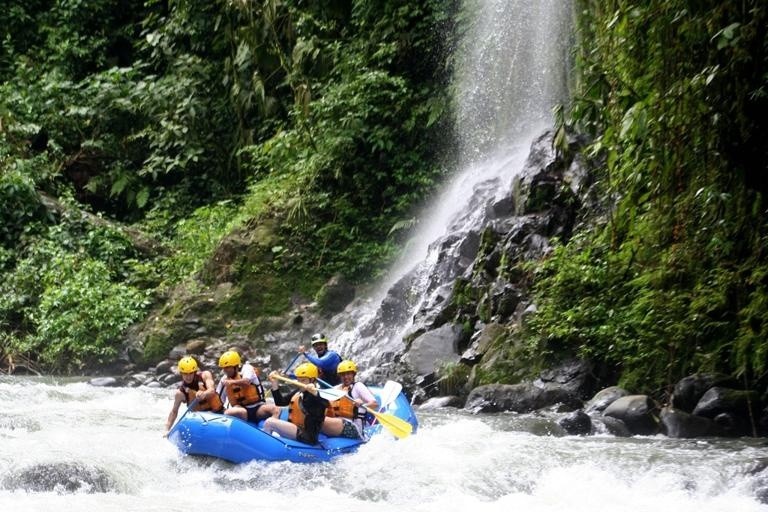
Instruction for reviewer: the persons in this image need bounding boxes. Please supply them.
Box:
[278,333,343,389]
[262,362,331,446]
[316,359,379,439]
[162,354,249,440]
[215,350,283,421]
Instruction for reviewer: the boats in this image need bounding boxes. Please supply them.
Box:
[170,382,418,465]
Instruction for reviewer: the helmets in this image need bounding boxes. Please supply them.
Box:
[294,363,318,384]
[178,355,198,374]
[336,360,358,376]
[218,351,242,369]
[311,333,328,345]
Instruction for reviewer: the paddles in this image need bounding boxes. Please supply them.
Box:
[273,373,348,402]
[315,377,413,441]
